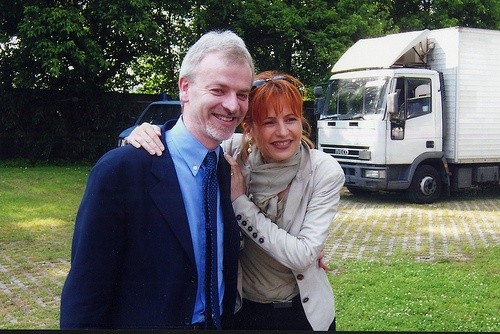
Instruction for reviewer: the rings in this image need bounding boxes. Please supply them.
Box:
[231,172,233,175]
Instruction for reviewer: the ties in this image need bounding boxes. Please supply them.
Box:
[200,151,221,332]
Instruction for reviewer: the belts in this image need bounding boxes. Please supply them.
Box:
[242,296,301,309]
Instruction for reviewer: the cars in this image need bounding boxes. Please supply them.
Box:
[116,101,182,148]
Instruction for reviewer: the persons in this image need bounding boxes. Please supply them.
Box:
[123,70,345,332]
[59,30,330,331]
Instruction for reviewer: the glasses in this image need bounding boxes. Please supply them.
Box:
[250,75,304,98]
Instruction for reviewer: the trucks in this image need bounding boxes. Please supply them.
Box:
[314,26,500,204]
